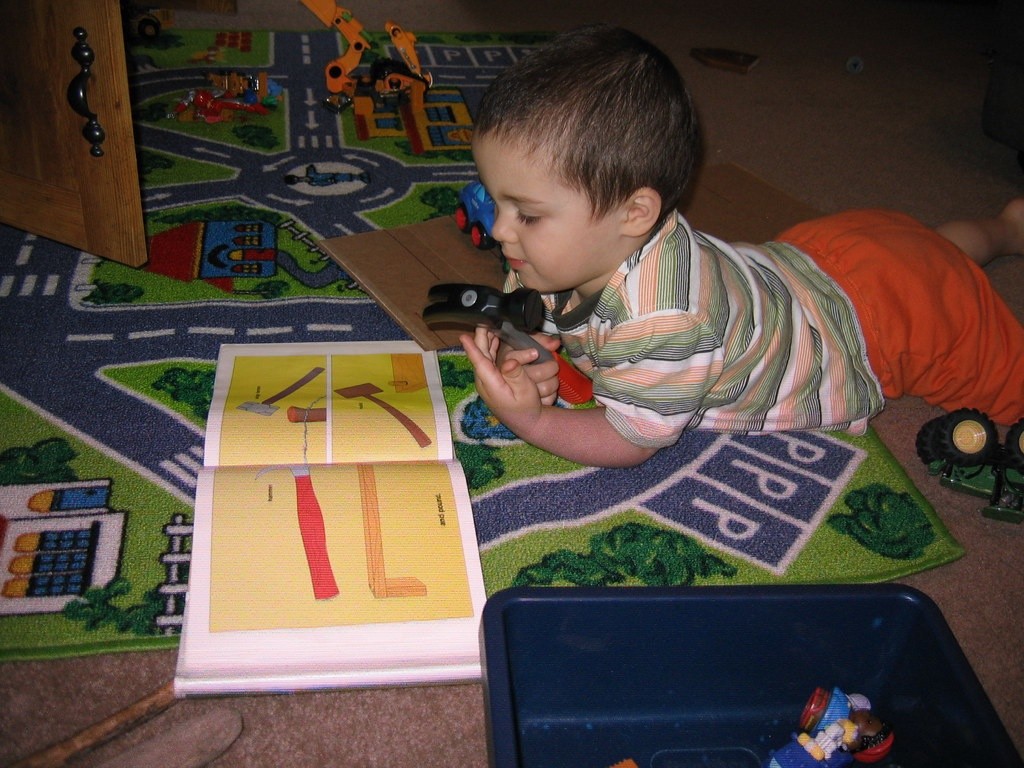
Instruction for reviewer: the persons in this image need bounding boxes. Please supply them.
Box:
[459,23,1024,471]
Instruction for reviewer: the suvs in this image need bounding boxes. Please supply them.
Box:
[915,407,1024,525]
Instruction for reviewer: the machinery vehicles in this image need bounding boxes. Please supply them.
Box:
[299,0,434,114]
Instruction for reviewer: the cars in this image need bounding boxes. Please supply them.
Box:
[456,179,498,250]
[128,7,176,40]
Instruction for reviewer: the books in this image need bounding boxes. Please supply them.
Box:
[175,340,490,698]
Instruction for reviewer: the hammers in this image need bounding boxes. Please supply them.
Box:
[423,282,597,404]
